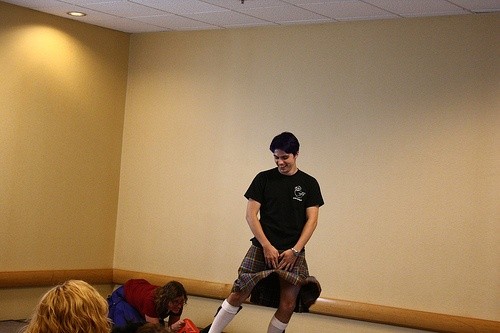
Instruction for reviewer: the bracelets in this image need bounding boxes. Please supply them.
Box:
[169,324,176,332]
[290,247,300,254]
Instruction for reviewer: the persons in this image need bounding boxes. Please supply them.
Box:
[106,274,188,333]
[20,279,113,333]
[207,131,325,333]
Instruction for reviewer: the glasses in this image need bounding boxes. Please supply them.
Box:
[171,299,185,306]
